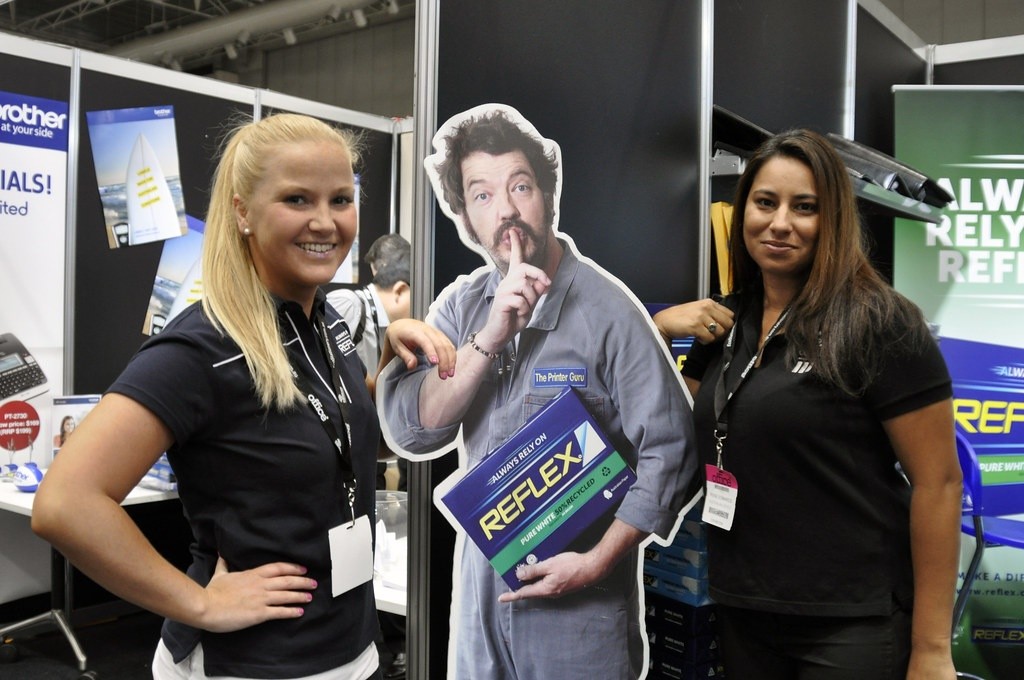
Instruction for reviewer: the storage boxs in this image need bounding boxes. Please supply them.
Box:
[441,385,635,592]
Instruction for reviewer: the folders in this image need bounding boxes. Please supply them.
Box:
[827,130,954,208]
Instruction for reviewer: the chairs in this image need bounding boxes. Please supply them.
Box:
[952,429,1024,639]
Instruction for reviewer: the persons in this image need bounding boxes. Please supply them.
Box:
[384,111,699,679]
[324,232,410,391]
[31,114,456,680]
[652,128,963,679]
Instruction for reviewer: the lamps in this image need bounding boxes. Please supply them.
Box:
[283,28,297,44]
[325,4,341,23]
[384,0,400,16]
[224,42,238,59]
[235,28,252,48]
[352,8,367,28]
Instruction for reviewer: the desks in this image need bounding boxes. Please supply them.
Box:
[0,467,180,680]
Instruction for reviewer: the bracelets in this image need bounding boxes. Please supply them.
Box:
[467,332,506,359]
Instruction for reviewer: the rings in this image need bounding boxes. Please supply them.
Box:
[708,321,717,332]
